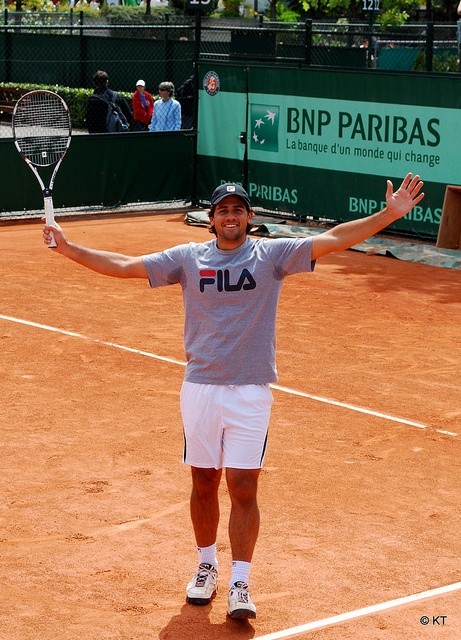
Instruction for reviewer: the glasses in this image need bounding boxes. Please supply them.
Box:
[158,88,165,92]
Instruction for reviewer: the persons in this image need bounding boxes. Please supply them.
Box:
[131,79,154,131]
[360,39,375,61]
[40,171,431,620]
[149,81,182,131]
[90,0,100,11]
[76,0,89,9]
[86,70,131,209]
[140,0,148,7]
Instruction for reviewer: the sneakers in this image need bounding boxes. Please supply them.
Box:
[227,581,256,619]
[186,563,219,605]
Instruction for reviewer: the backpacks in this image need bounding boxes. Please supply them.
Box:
[90,91,130,132]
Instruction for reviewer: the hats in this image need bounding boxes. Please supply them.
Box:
[136,80,145,87]
[210,183,250,205]
